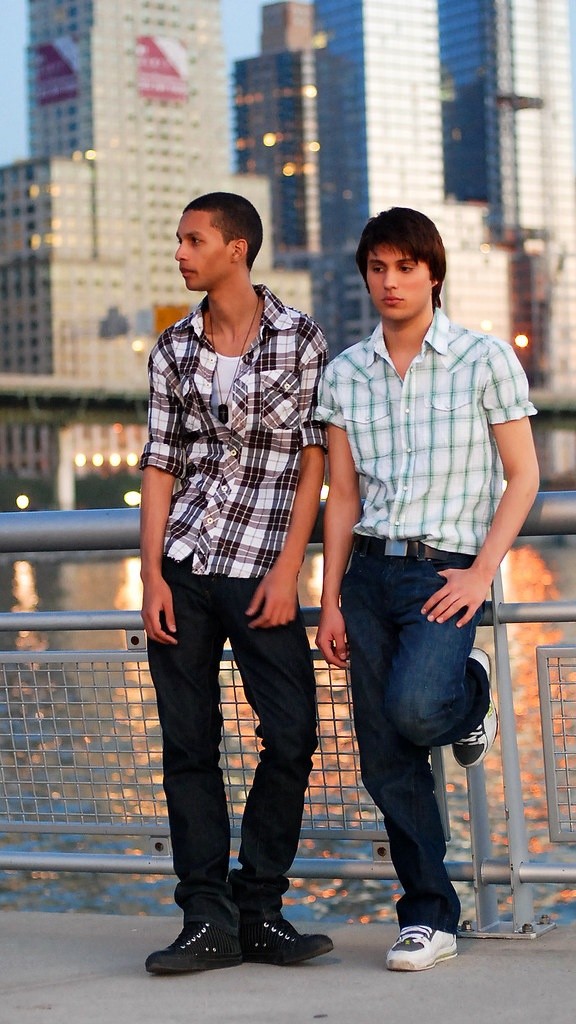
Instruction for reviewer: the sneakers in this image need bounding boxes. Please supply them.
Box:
[145,915,241,973]
[385,925,459,972]
[450,648,500,768]
[241,914,332,966]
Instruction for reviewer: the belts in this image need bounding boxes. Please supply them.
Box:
[355,535,449,561]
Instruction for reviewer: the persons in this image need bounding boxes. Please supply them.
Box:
[138,192,335,978]
[315,208,540,970]
